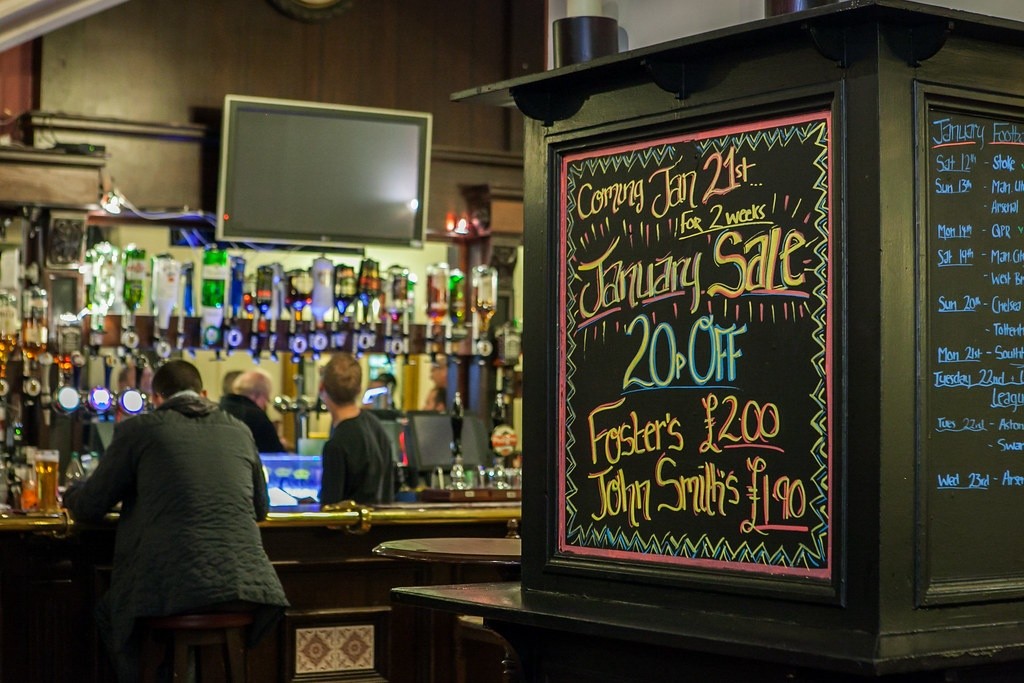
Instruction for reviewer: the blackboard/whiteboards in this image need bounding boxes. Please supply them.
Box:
[548,98,843,596]
[912,81,1024,609]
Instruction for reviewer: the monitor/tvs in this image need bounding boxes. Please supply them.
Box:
[215,95,435,249]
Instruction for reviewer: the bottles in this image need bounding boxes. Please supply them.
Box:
[450,457,465,488]
[86,449,101,479]
[474,264,499,340]
[494,459,505,488]
[21,290,47,367]
[493,393,506,417]
[0,292,17,376]
[155,250,467,332]
[452,392,464,416]
[62,451,84,487]
[89,240,120,333]
[125,245,145,334]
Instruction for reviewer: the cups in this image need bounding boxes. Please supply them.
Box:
[36,451,60,512]
[553,16,620,70]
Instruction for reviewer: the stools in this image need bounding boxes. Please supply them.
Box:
[165,612,254,683]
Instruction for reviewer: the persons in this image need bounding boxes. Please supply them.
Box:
[114,362,155,424]
[63,358,292,683]
[218,366,286,453]
[423,384,462,443]
[367,372,398,410]
[316,353,394,506]
